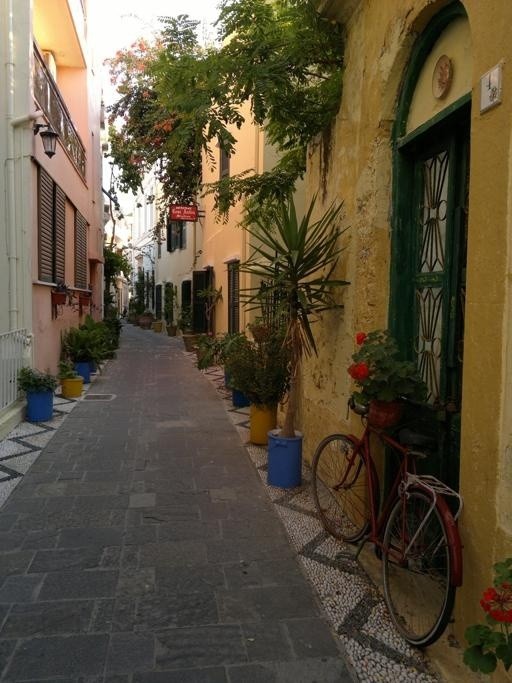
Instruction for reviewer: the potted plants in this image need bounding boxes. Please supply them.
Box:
[19,366,59,422]
[50,279,222,399]
[220,188,352,490]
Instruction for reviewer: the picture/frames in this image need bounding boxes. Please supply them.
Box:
[478,60,503,116]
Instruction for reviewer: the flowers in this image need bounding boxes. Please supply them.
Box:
[345,327,434,403]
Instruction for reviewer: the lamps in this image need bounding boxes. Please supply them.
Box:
[33,121,60,158]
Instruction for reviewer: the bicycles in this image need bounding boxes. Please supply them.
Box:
[311,390,468,652]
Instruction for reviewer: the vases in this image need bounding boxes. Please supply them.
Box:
[366,397,402,430]
